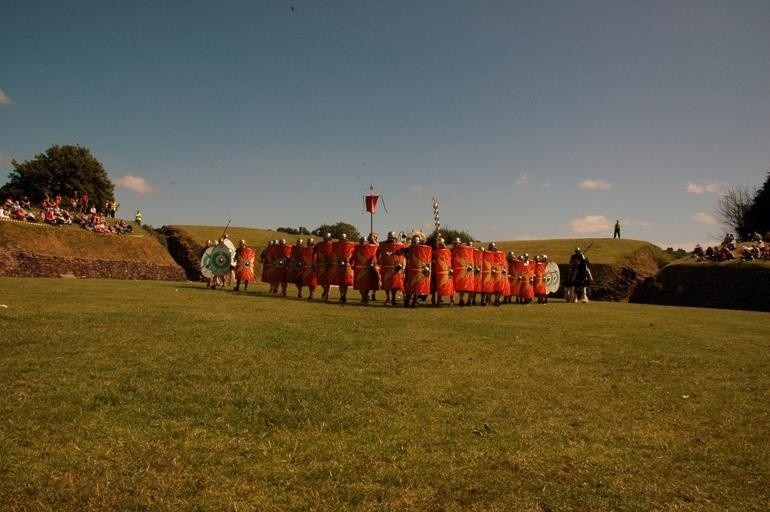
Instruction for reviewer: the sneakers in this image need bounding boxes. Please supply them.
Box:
[207,284,224,289]
[502,298,549,304]
[234,286,248,291]
[383,298,419,307]
[269,288,287,297]
[298,294,313,299]
[338,296,348,302]
[361,294,376,304]
[431,300,455,308]
[459,300,501,307]
[322,292,329,300]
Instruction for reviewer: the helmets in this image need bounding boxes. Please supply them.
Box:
[436,238,445,244]
[388,232,396,238]
[508,251,547,261]
[488,242,497,249]
[324,232,331,238]
[296,239,303,243]
[359,236,368,242]
[575,247,581,252]
[453,237,474,246]
[269,239,286,245]
[205,235,247,246]
[412,236,419,243]
[339,233,347,238]
[307,238,315,243]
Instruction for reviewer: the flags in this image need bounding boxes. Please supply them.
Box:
[363,194,381,215]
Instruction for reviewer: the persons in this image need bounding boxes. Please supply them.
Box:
[258,231,552,309]
[232,239,255,291]
[2,189,133,236]
[134,209,143,228]
[222,232,238,286]
[212,239,222,286]
[198,238,219,287]
[210,237,230,290]
[613,219,621,239]
[691,229,770,264]
[568,247,594,284]
